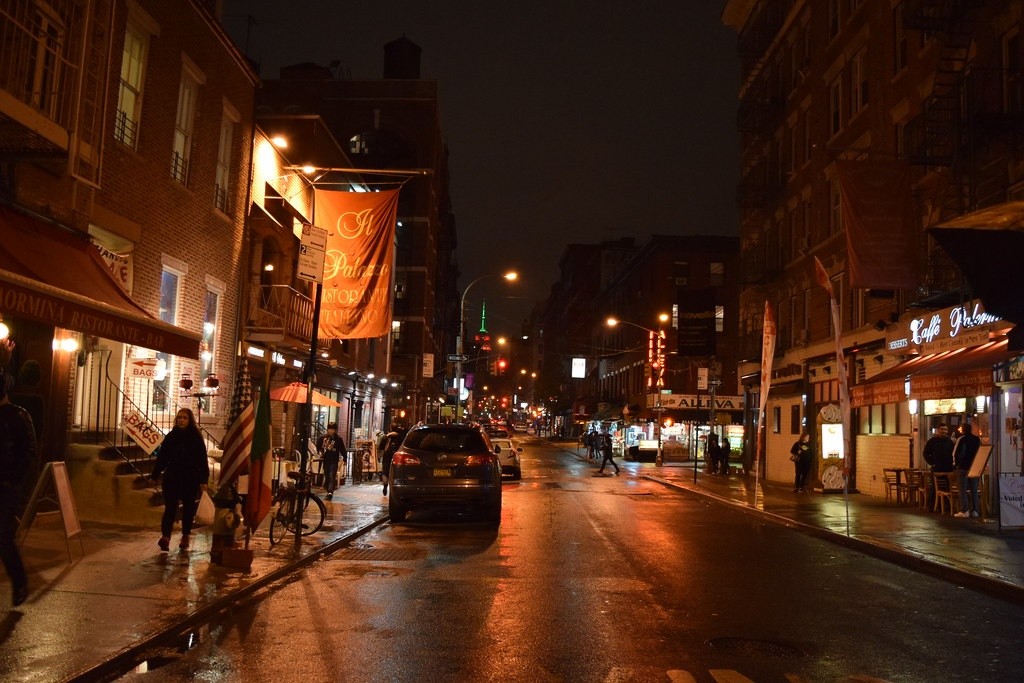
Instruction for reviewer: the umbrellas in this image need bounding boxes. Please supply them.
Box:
[270,381,343,407]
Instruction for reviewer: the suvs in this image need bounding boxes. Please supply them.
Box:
[386,420,504,526]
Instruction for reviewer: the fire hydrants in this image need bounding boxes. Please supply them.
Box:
[209,481,249,566]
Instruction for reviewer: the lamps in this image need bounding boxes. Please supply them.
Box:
[874,319,888,331]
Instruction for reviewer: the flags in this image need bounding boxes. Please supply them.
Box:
[218,356,273,534]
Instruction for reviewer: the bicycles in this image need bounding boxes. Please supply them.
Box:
[266,459,327,545]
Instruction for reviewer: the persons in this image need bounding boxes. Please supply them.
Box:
[149,407,209,552]
[723,438,730,472]
[13,360,45,438]
[583,430,602,459]
[0,375,40,606]
[597,437,620,474]
[790,433,812,492]
[379,422,399,496]
[952,423,982,517]
[561,426,565,438]
[709,439,721,474]
[923,423,955,513]
[317,422,348,500]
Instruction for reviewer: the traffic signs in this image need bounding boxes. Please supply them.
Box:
[297,222,332,292]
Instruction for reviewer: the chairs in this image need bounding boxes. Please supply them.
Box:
[881,467,988,515]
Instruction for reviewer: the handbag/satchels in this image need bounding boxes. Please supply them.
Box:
[790,442,800,462]
[194,491,215,524]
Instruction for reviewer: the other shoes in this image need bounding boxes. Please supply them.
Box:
[383,483,388,496]
[792,488,797,492]
[158,534,169,551]
[800,489,806,492]
[179,536,190,548]
[615,469,620,474]
[971,510,978,517]
[597,470,603,473]
[325,491,332,500]
[13,580,28,604]
[954,510,969,518]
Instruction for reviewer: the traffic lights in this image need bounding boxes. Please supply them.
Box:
[499,360,506,376]
[447,353,470,365]
[489,361,497,377]
[707,379,724,386]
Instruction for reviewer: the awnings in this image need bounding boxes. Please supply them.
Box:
[849,335,1022,408]
[0,204,203,361]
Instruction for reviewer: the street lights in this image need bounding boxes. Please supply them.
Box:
[605,315,666,466]
[452,269,521,420]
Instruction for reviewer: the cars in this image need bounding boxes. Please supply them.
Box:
[515,421,529,432]
[488,438,527,479]
[482,418,512,440]
